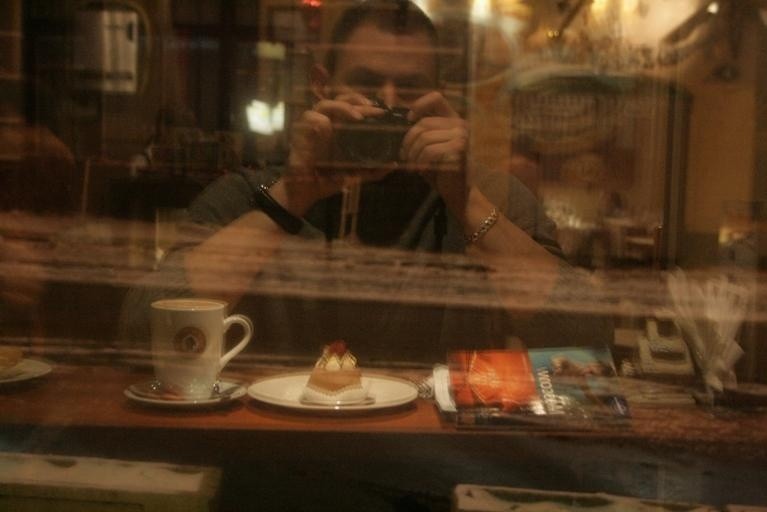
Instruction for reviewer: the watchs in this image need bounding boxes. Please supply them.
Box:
[249,184,304,235]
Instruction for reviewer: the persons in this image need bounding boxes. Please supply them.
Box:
[118,0,615,363]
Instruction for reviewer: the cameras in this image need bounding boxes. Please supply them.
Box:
[317,107,462,173]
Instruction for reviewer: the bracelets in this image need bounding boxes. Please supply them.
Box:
[469,207,499,247]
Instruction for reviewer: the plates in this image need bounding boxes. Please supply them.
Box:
[248,370,420,414]
[1,347,54,385]
[125,382,248,407]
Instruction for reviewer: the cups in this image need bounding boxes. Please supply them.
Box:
[147,297,254,401]
